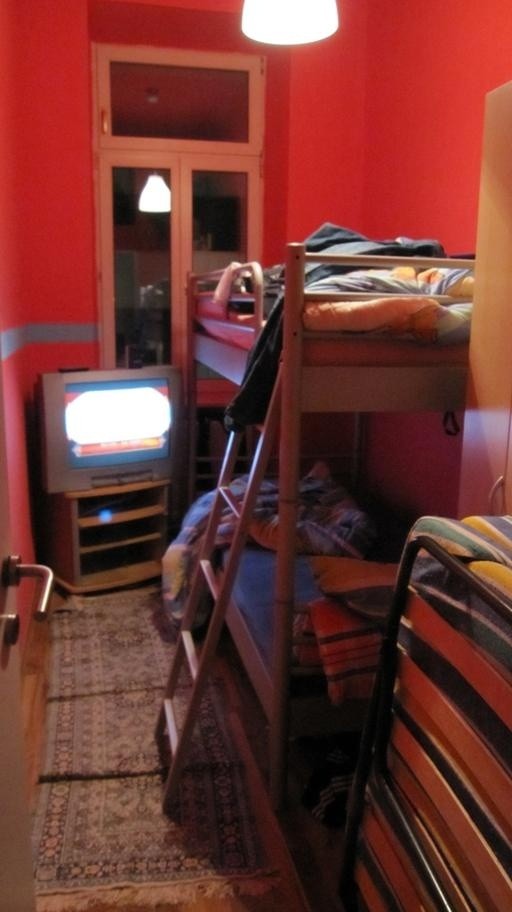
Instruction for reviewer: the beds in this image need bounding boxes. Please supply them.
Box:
[189,254,476,413]
[183,456,407,809]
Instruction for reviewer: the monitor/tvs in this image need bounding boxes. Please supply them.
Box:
[39,366,188,493]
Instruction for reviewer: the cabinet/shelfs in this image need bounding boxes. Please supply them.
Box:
[51,480,170,595]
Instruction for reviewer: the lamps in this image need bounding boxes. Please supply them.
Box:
[237,1,344,46]
[137,168,174,212]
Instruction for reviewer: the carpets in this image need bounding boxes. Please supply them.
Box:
[25,590,276,899]
